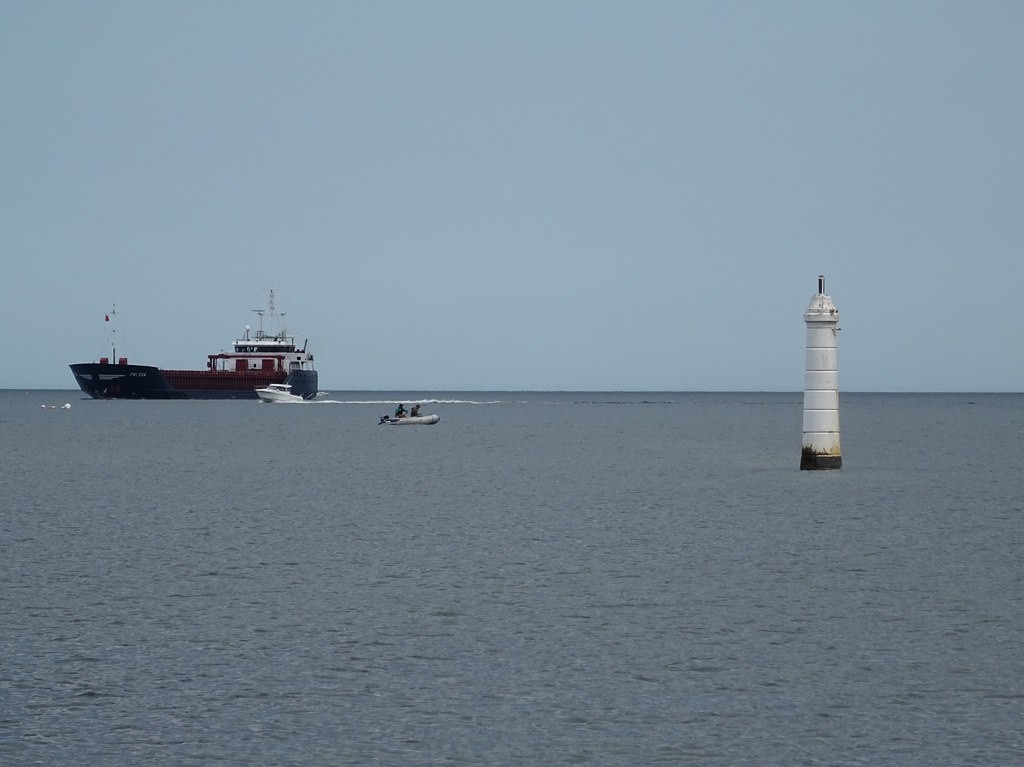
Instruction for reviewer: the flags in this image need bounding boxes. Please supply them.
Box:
[105,314,109,322]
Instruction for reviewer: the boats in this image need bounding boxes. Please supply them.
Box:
[67,288,317,402]
[255,382,304,403]
[378,414,440,425]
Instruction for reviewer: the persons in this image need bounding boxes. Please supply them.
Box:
[411,404,421,417]
[395,403,409,420]
